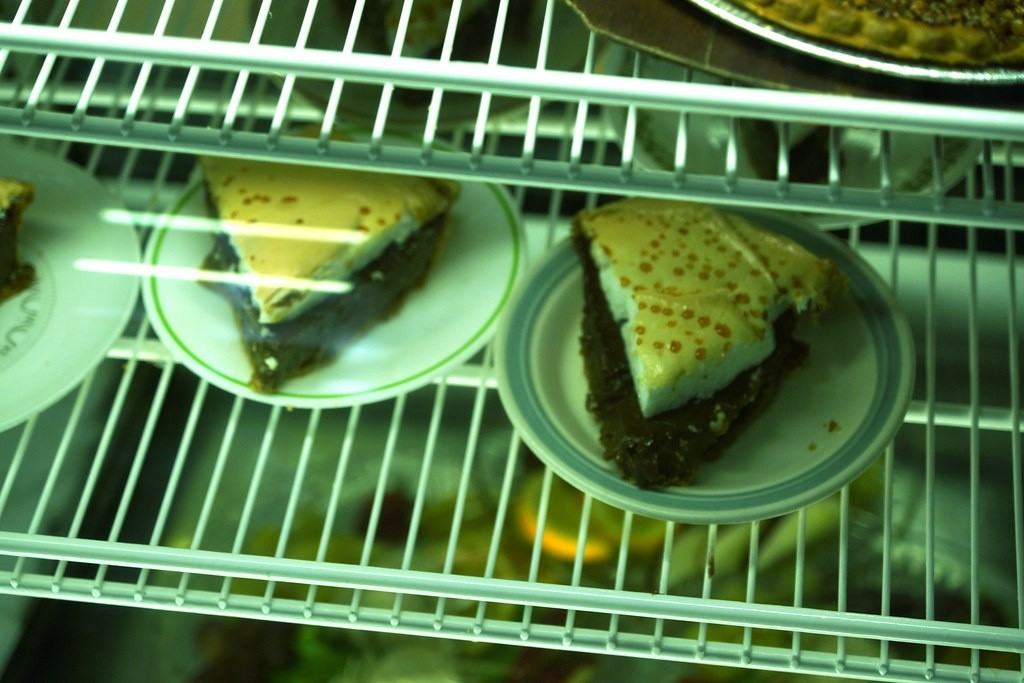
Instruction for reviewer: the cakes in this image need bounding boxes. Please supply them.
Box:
[195,124,470,391]
[728,0,1024,73]
[570,198,840,488]
[0,176,37,302]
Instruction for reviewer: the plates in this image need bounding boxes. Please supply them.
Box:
[492,209,917,523]
[0,137,141,433]
[594,43,982,230]
[247,1,586,126]
[685,0,1024,83]
[141,134,528,404]
